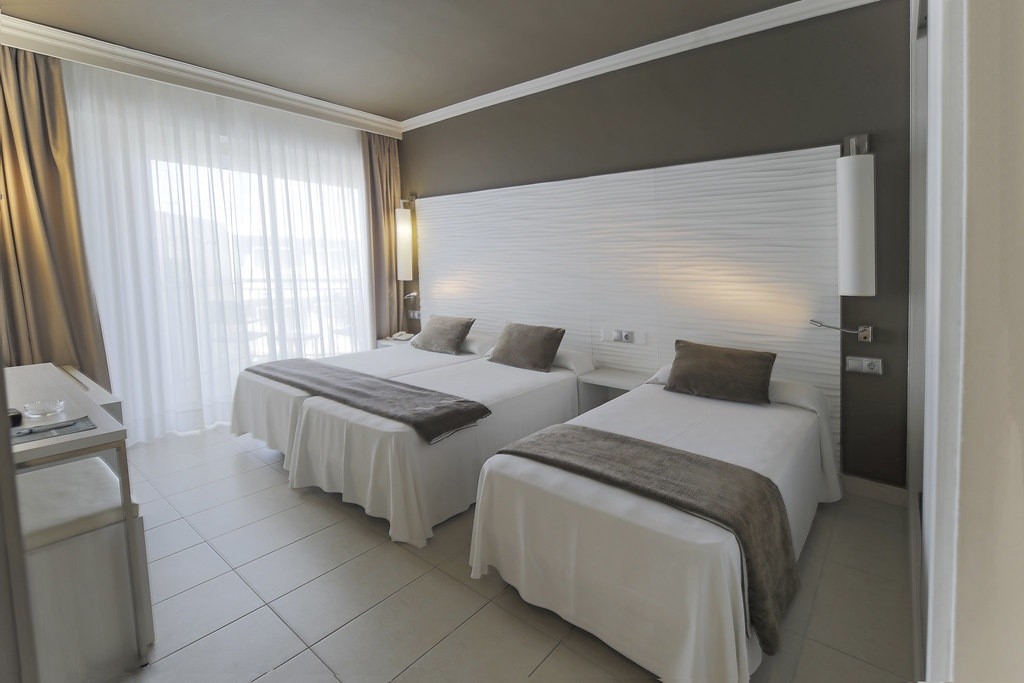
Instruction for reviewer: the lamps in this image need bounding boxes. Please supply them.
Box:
[836,135,876,296]
[395,199,413,281]
[809,320,873,343]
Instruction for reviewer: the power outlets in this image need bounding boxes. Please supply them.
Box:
[621,331,634,343]
[863,359,884,376]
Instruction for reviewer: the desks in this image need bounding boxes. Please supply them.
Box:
[23,364,124,478]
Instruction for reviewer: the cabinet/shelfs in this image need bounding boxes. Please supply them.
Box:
[4,363,156,683]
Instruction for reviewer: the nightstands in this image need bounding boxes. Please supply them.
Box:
[576,366,654,415]
[377,335,413,349]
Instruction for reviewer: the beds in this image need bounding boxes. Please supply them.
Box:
[470,338,845,683]
[289,322,599,547]
[230,314,496,472]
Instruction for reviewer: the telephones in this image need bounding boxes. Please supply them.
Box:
[392,331,414,341]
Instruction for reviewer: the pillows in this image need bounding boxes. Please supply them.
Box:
[410,315,477,356]
[487,322,566,373]
[663,340,776,405]
